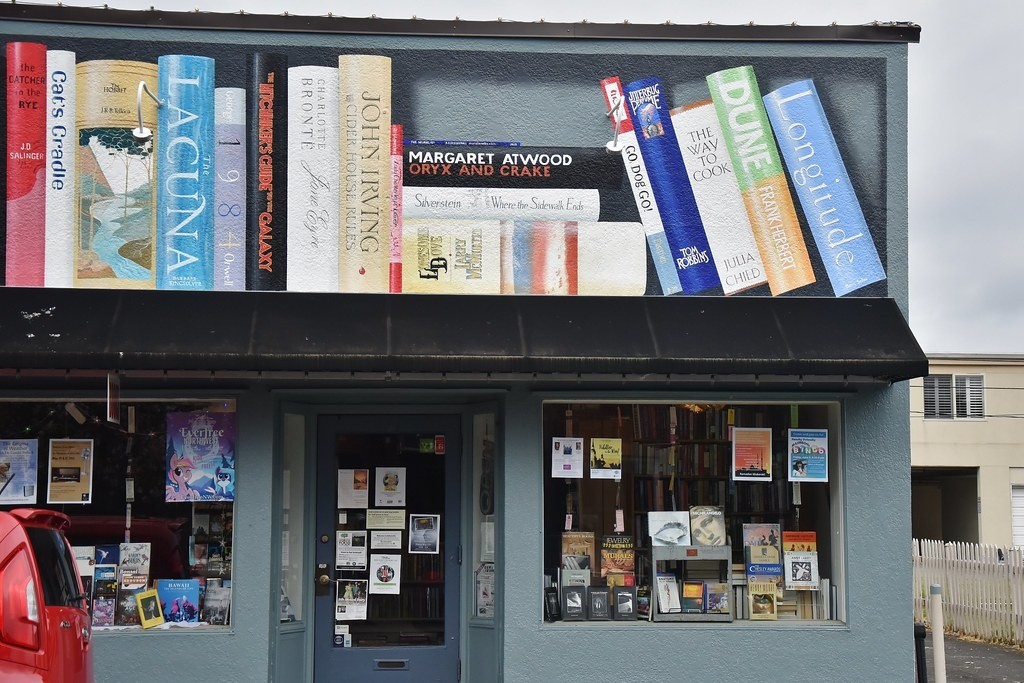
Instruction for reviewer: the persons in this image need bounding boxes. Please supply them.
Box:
[182,596,198,618]
[169,599,180,622]
[690,506,726,546]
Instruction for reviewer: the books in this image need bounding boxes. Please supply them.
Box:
[72,541,230,627]
[336,512,445,646]
[543,400,838,622]
[4,40,886,298]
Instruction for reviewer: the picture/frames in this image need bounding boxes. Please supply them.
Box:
[137,589,165,628]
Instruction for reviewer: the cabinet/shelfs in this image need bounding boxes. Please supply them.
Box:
[630,423,795,554]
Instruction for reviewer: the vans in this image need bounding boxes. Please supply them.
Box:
[64,512,189,582]
[0,507,94,683]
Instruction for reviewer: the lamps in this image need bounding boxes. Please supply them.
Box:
[605,95,626,156]
[132,80,163,143]
